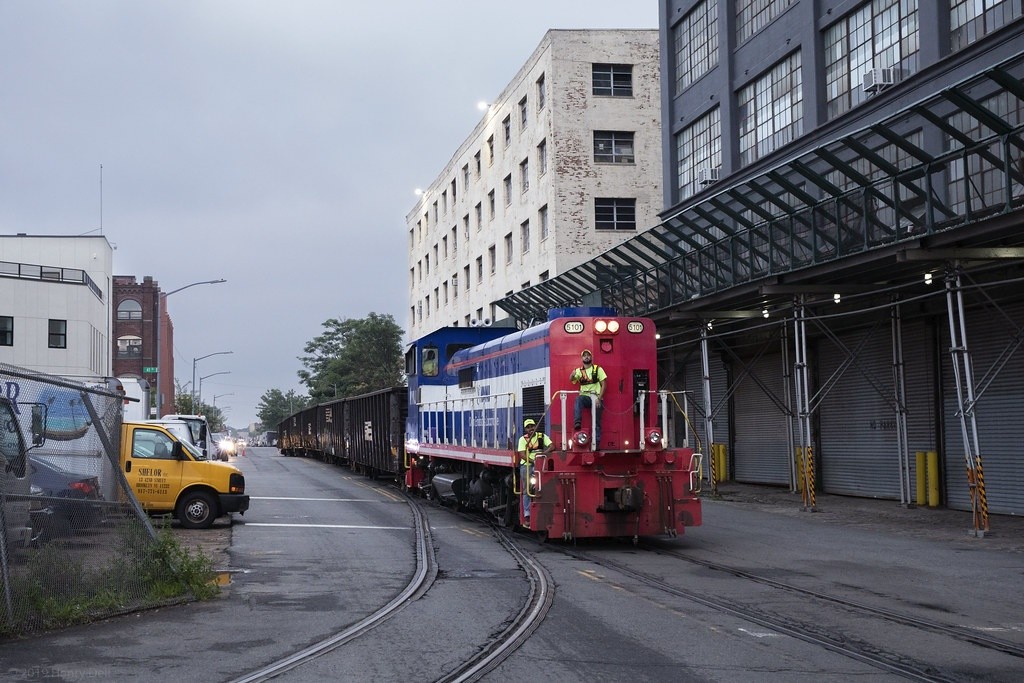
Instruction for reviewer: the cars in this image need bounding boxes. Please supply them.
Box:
[0,444,108,540]
[145,413,246,463]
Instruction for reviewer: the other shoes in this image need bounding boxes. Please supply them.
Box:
[523,515,530,522]
[574,424,581,430]
[596,445,600,451]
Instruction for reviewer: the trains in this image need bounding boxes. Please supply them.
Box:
[274,303,705,552]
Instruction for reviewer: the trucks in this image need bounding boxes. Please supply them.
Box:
[0,372,251,532]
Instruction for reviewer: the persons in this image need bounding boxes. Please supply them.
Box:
[517,419,555,527]
[569,349,607,451]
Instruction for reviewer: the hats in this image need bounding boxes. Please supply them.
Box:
[581,349,591,356]
[524,418,535,427]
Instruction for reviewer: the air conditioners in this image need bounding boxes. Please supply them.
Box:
[699,168,718,183]
[465,232,469,238]
[452,279,458,286]
[862,67,893,91]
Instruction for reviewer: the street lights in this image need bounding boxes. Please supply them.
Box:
[191,351,234,416]
[212,393,235,432]
[156,278,227,419]
[199,370,232,416]
